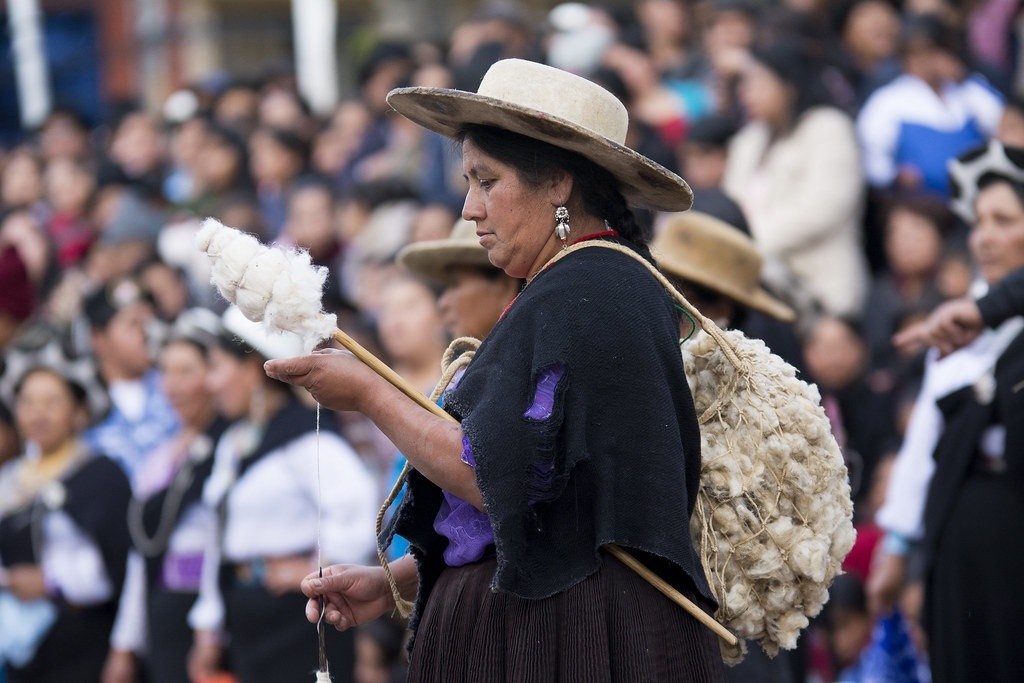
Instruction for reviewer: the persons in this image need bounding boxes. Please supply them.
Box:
[264,60,727,683]
[0,0,1024,683]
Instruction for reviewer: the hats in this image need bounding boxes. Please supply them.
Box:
[647,209,798,321]
[385,57,694,213]
[84,279,154,331]
[222,301,313,362]
[162,306,222,351]
[395,216,492,285]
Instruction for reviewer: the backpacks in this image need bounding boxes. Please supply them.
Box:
[377,241,856,666]
